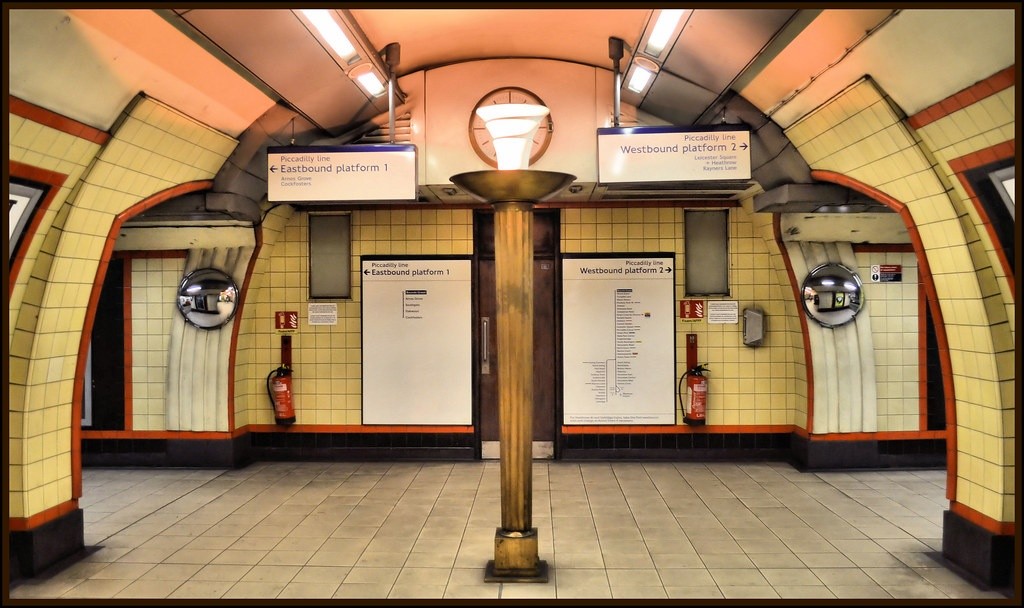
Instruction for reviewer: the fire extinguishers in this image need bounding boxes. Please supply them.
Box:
[266,363,296,425]
[678,363,711,427]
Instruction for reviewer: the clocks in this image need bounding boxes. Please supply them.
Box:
[468,87,553,169]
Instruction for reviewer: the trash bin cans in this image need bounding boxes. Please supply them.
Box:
[814,295,819,305]
